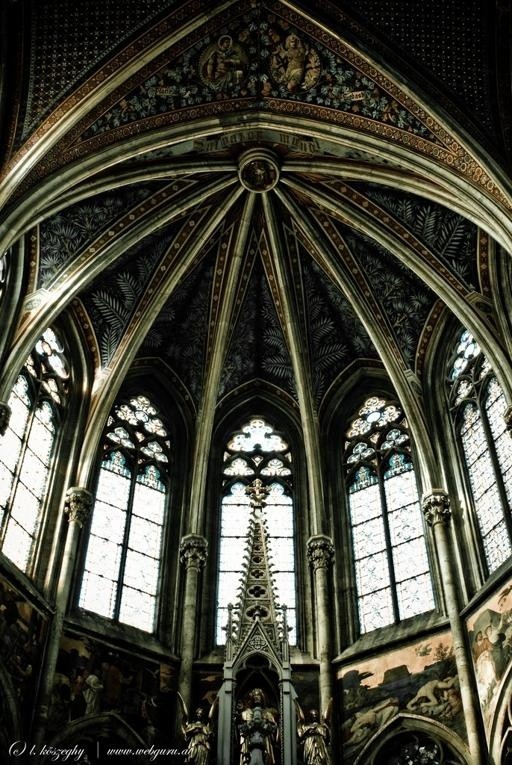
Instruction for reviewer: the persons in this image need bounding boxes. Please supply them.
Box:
[468,631,498,701]
[235,686,282,764]
[174,686,223,764]
[295,697,334,765]
